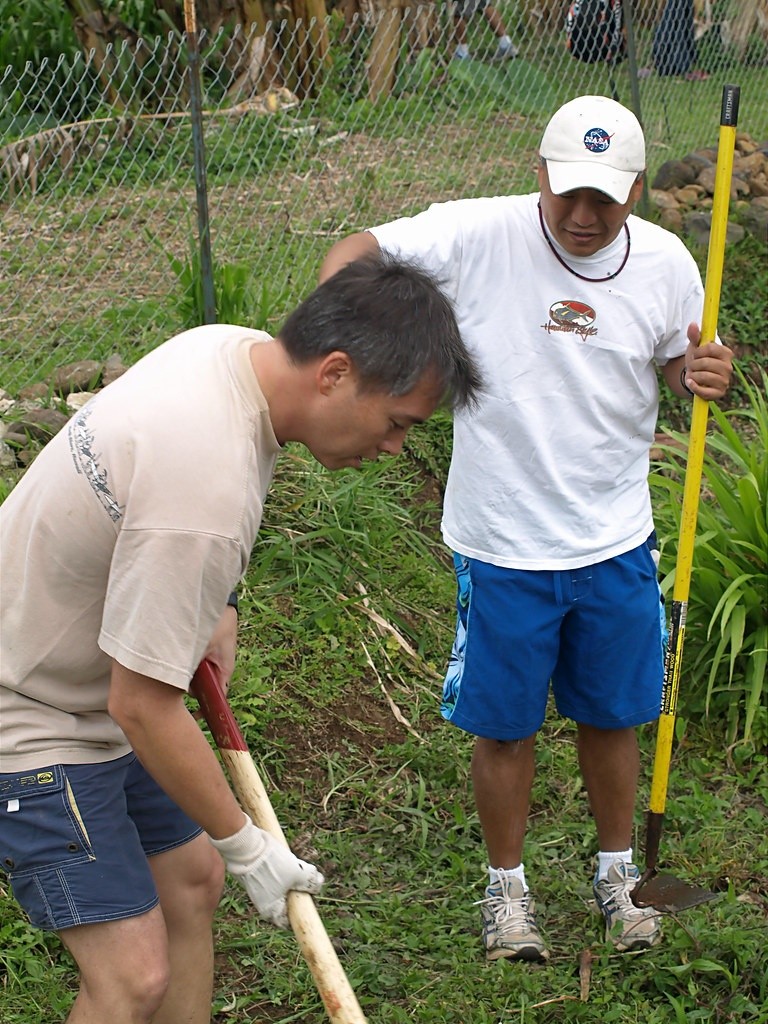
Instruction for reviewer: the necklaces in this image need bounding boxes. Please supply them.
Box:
[536,196,632,284]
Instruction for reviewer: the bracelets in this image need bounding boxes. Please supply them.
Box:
[227,591,240,616]
[679,366,694,399]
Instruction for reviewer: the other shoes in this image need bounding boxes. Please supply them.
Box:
[444,48,473,63]
[492,46,520,60]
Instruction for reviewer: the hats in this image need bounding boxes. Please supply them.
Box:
[538,94,647,204]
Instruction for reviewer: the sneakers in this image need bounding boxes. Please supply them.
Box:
[594,858,664,950]
[473,867,551,961]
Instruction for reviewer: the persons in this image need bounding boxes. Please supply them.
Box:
[449,0,522,69]
[0,241,492,1024]
[570,0,712,81]
[312,93,738,966]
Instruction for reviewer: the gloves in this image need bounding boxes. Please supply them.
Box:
[210,810,325,931]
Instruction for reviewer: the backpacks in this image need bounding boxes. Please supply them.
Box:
[565,0,623,64]
[653,0,699,75]
